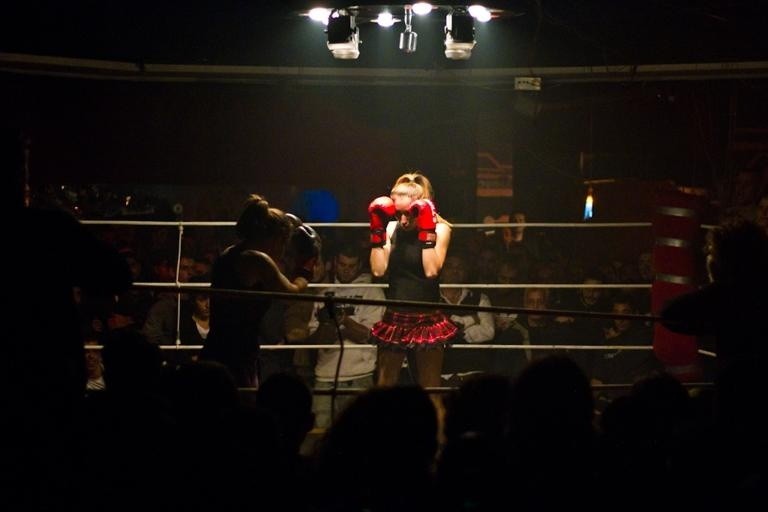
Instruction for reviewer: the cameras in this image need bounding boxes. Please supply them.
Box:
[315,291,356,323]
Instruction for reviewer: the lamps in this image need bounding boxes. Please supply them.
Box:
[298,0,504,60]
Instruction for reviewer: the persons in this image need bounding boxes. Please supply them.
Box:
[1,168,767,512]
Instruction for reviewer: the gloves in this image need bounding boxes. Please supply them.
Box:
[367,195,397,247]
[405,198,438,248]
[285,213,303,229]
[293,223,322,281]
